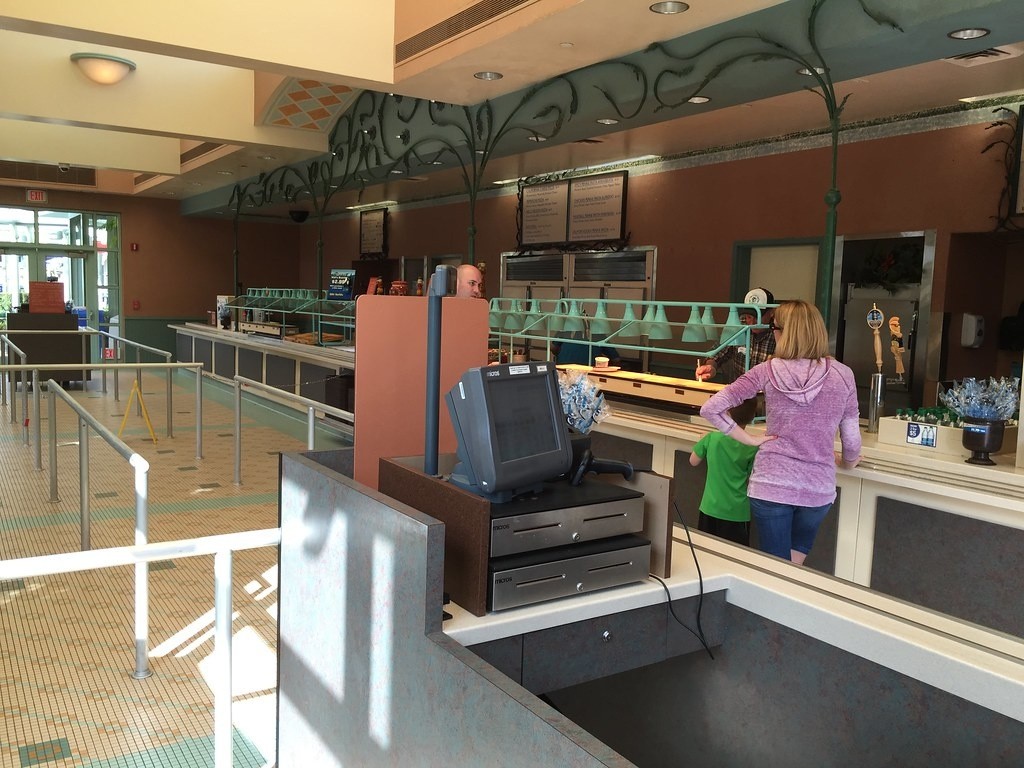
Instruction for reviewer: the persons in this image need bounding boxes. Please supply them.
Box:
[700,298,863,566]
[551,322,621,365]
[697,287,776,416]
[889,317,906,374]
[689,397,760,546]
[457,264,483,298]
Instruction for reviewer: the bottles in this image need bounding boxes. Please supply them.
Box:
[921,427,928,444]
[895,403,1020,427]
[928,426,934,446]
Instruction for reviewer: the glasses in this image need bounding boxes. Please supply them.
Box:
[769,322,781,332]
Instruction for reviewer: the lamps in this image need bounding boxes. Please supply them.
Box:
[71,52,136,84]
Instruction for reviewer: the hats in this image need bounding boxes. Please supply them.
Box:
[737,286,774,318]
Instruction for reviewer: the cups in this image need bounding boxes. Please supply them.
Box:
[595,357,609,368]
[513,353,527,363]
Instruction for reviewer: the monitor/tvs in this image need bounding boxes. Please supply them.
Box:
[444,361,573,504]
